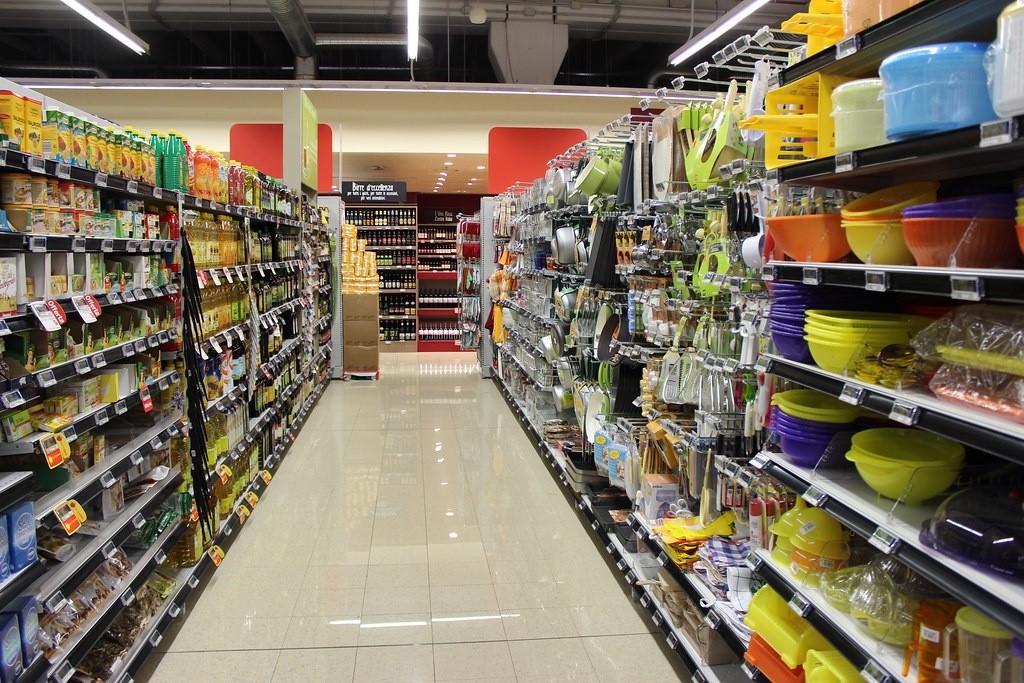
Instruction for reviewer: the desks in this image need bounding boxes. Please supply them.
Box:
[343,292,380,380]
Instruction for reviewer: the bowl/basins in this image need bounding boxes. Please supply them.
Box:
[767,388,970,507]
[764,181,1024,269]
[766,281,828,363]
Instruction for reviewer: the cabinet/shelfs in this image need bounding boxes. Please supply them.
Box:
[475,0,1024,683]
[0,73,347,683]
[343,205,463,353]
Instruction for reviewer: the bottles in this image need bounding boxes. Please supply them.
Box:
[418,287,459,308]
[418,226,457,240]
[378,272,416,289]
[378,295,416,317]
[104,125,300,222]
[418,242,458,255]
[379,321,417,342]
[355,230,416,246]
[419,320,462,341]
[418,258,458,273]
[103,197,301,269]
[376,250,416,266]
[195,266,303,343]
[168,350,316,568]
[160,304,300,410]
[345,207,415,227]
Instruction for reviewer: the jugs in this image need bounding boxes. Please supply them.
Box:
[902,597,1024,683]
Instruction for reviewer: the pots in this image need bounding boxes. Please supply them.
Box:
[535,145,627,451]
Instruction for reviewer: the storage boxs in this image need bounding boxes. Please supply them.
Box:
[0,501,38,585]
[0,89,44,159]
[0,375,106,467]
[0,596,40,683]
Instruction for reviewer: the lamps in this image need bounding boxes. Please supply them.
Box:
[59,1,150,56]
[666,0,769,67]
[469,0,487,25]
